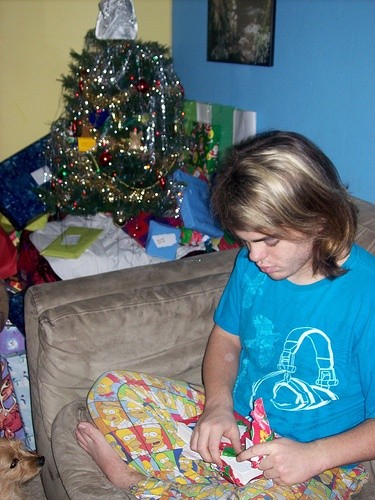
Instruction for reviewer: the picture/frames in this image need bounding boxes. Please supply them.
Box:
[207,0,277,66]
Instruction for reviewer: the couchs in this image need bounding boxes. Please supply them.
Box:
[23,193,375,500]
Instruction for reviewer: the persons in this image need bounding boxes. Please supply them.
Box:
[76,129,374,500]
